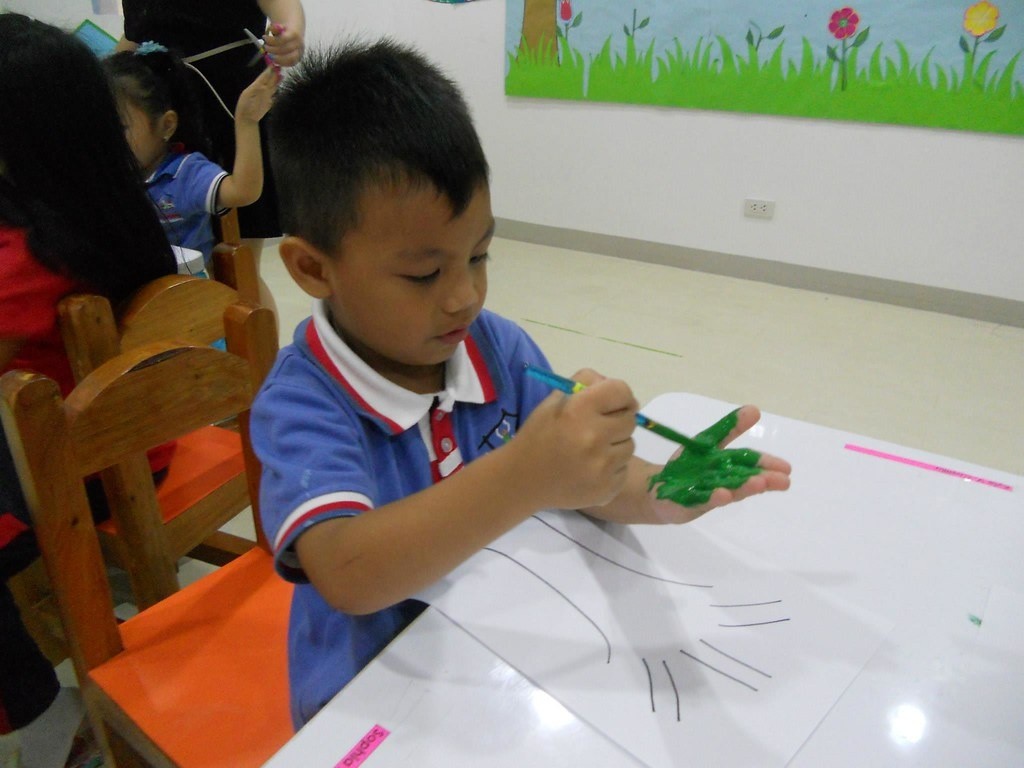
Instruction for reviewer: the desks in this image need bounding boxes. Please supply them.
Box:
[170,243,209,280]
[263,394,1024,768]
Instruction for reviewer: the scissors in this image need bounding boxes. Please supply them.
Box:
[244,23,284,74]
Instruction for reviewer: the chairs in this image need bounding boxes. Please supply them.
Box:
[0,302,354,768]
[54,202,261,613]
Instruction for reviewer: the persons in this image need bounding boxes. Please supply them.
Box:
[249,41,791,734]
[0,12,177,532]
[97,44,281,352]
[115,0,305,243]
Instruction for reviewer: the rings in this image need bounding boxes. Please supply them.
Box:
[274,63,280,68]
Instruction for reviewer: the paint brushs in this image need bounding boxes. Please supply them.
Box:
[523,363,711,455]
